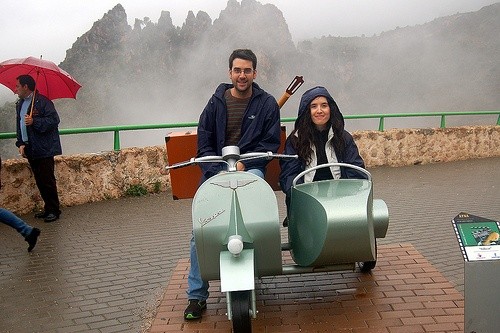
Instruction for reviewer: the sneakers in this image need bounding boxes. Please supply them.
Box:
[184,299,207,319]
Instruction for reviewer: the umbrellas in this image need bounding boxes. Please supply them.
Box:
[0,55,82,117]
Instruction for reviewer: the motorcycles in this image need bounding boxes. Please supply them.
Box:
[165,144,390,333]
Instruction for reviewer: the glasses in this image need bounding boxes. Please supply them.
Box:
[233,68,255,74]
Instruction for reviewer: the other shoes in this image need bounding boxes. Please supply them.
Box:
[25,228,40,251]
[35,210,59,221]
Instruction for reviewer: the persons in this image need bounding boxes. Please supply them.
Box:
[279,86,368,227]
[15,75,62,222]
[0,208,40,252]
[184,48,281,320]
